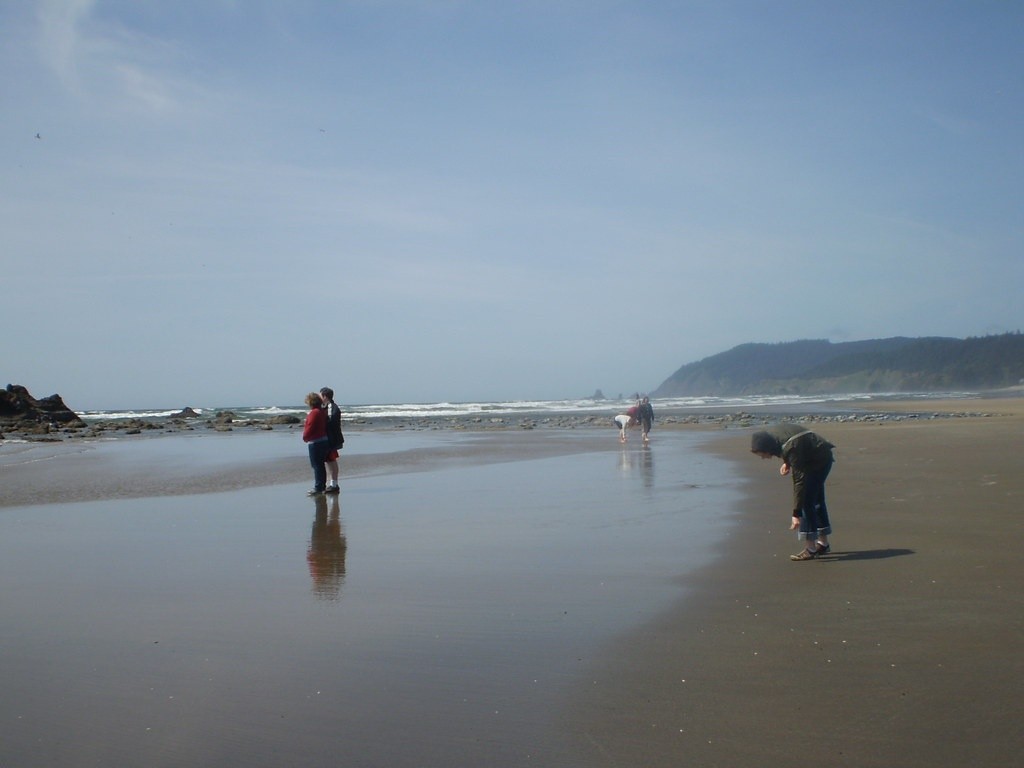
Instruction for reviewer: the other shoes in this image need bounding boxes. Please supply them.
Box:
[326,484,340,492]
[307,489,323,496]
[789,548,820,560]
[644,438,650,442]
[814,542,831,554]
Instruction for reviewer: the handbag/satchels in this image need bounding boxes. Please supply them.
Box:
[815,433,837,449]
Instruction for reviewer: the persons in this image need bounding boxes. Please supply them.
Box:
[613,396,654,443]
[303,388,344,494]
[750,424,835,561]
